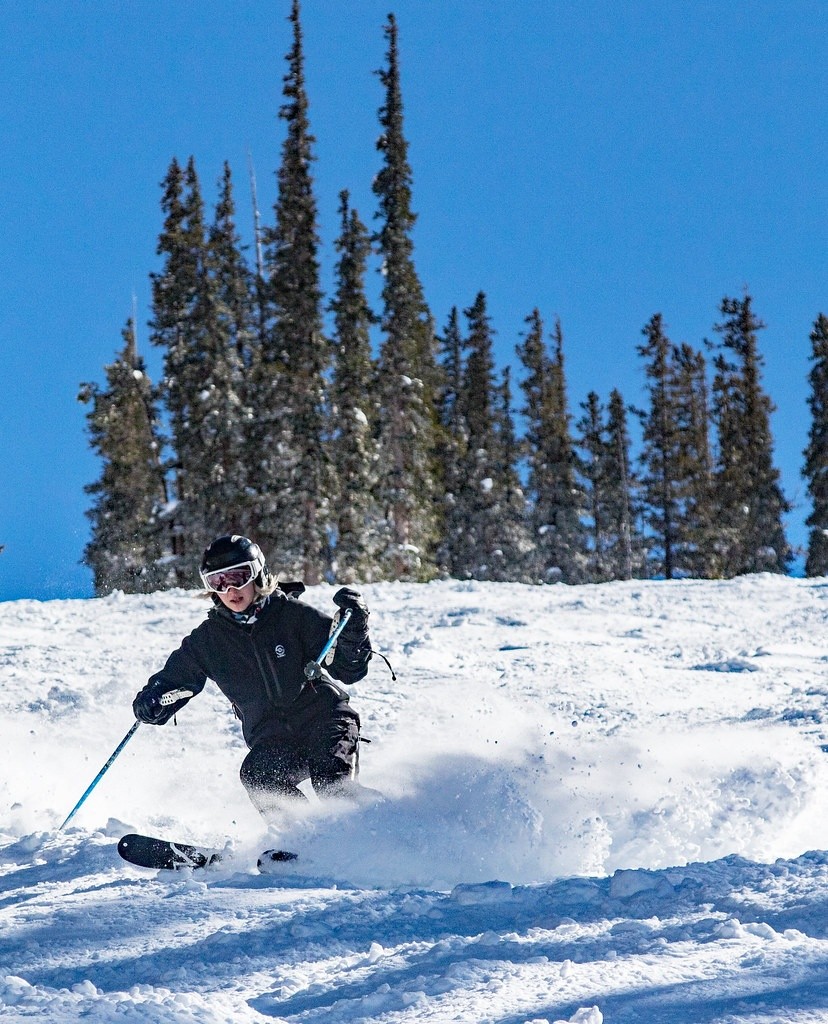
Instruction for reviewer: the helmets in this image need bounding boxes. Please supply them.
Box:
[199,535,265,598]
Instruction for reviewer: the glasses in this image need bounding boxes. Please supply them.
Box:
[204,560,262,594]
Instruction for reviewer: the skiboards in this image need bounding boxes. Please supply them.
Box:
[116,831,316,877]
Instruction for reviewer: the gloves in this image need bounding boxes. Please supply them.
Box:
[133,689,165,725]
[333,587,369,627]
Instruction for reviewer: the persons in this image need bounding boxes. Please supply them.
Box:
[132,535,397,874]
